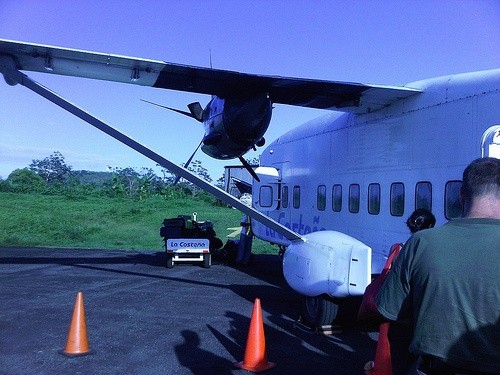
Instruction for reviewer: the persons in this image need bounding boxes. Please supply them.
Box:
[388,208,437,375]
[372,157,500,375]
[233,214,254,268]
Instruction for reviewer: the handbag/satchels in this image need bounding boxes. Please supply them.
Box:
[355,242,401,333]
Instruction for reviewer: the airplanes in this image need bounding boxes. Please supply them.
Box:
[1,38,500,329]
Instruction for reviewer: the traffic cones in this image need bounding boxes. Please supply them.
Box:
[58,291,97,357]
[233,298,276,372]
[364,323,394,374]
